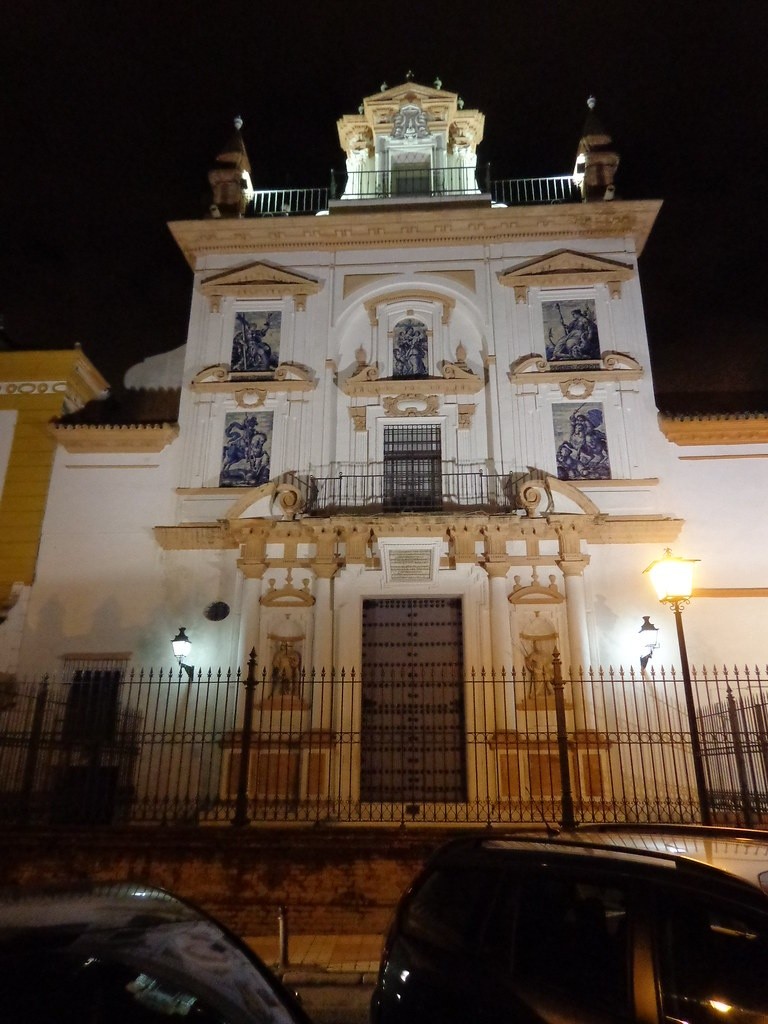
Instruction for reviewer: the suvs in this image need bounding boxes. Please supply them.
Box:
[370,824,768,1024]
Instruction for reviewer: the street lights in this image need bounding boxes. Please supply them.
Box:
[643,547,712,827]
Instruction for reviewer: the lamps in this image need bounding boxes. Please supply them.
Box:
[639,616,660,668]
[171,627,195,680]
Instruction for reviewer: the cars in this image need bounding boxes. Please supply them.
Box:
[0,881,313,1024]
